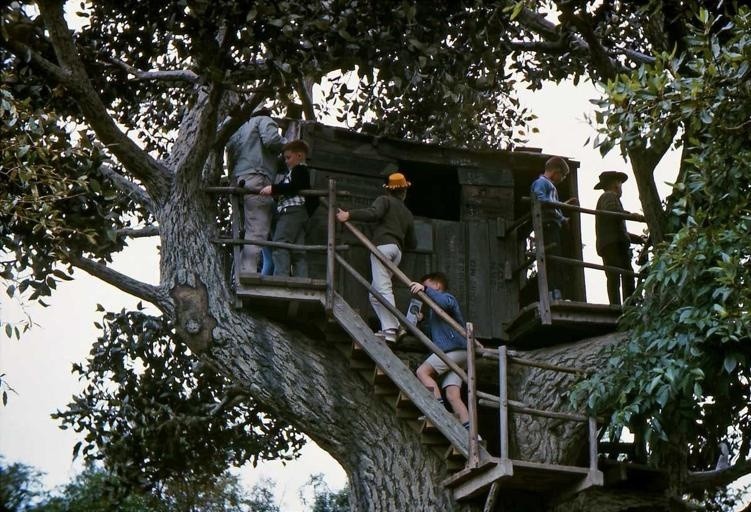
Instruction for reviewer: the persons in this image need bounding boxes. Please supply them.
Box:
[335,169,419,343]
[260,137,320,279]
[225,108,294,275]
[410,272,486,443]
[531,157,579,304]
[594,170,645,307]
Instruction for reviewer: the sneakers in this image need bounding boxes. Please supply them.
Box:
[397,325,408,342]
[374,327,397,343]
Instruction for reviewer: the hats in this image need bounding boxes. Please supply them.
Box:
[382,172,412,190]
[594,169,629,190]
